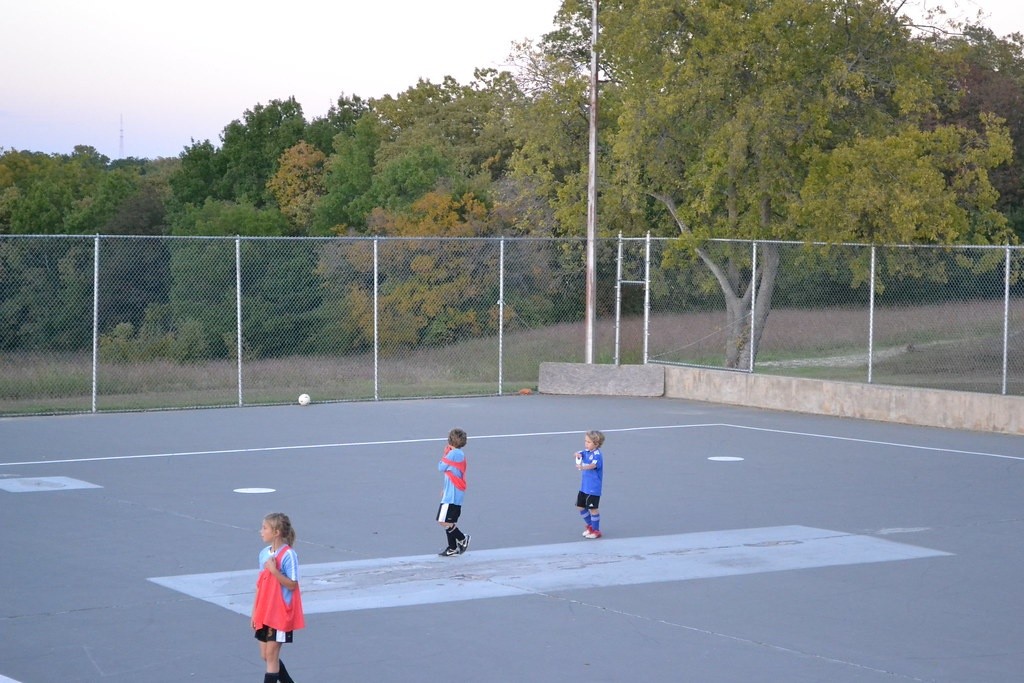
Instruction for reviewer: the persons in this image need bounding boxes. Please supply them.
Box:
[250,512,305,683]
[575,430,605,538]
[436,428,471,557]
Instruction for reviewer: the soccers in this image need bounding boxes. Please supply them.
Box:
[298,394,311,406]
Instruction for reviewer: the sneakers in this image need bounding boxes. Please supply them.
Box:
[582,523,601,539]
[458,534,471,555]
[438,547,458,557]
